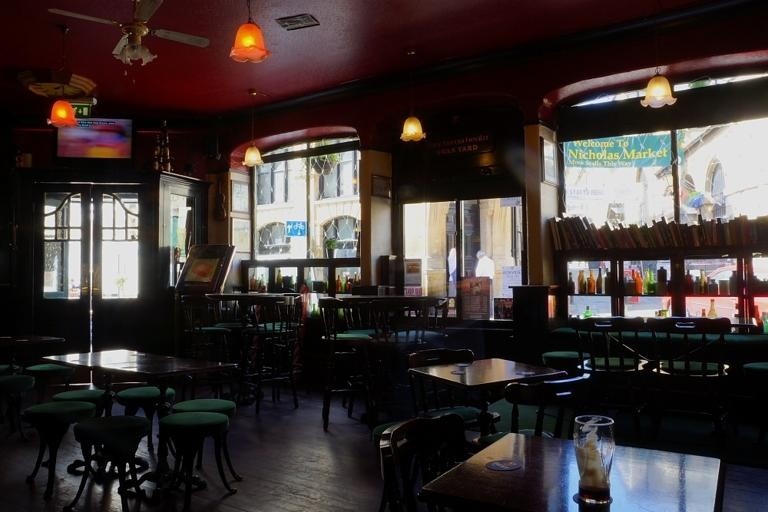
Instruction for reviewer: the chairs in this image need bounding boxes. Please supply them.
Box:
[0,290,766,512]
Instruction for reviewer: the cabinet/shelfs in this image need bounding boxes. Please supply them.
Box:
[551,248,767,319]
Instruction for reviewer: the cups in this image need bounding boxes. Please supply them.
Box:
[571,413,614,503]
[655,310,670,317]
[326,238,335,259]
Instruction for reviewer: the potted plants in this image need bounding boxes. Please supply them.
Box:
[306,137,340,175]
[323,235,338,259]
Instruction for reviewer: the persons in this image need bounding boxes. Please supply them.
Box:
[447,247,457,296]
[476,249,495,316]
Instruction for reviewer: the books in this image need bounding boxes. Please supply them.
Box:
[549,214,768,251]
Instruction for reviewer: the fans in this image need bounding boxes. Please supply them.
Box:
[48,0,214,53]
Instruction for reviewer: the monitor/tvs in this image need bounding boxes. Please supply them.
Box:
[180,255,224,284]
[56,116,136,160]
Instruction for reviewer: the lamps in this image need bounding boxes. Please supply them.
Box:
[634,0,684,112]
[47,74,81,132]
[398,50,430,146]
[228,0,273,65]
[238,86,270,170]
[110,31,157,86]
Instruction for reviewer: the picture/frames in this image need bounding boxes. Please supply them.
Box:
[539,134,561,190]
[228,217,252,255]
[228,179,253,215]
[370,173,391,200]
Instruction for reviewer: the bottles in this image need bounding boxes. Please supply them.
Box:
[752,305,768,335]
[631,267,667,296]
[583,306,592,318]
[700,298,717,320]
[567,264,609,295]
[249,267,361,295]
[310,303,320,317]
[684,268,739,297]
